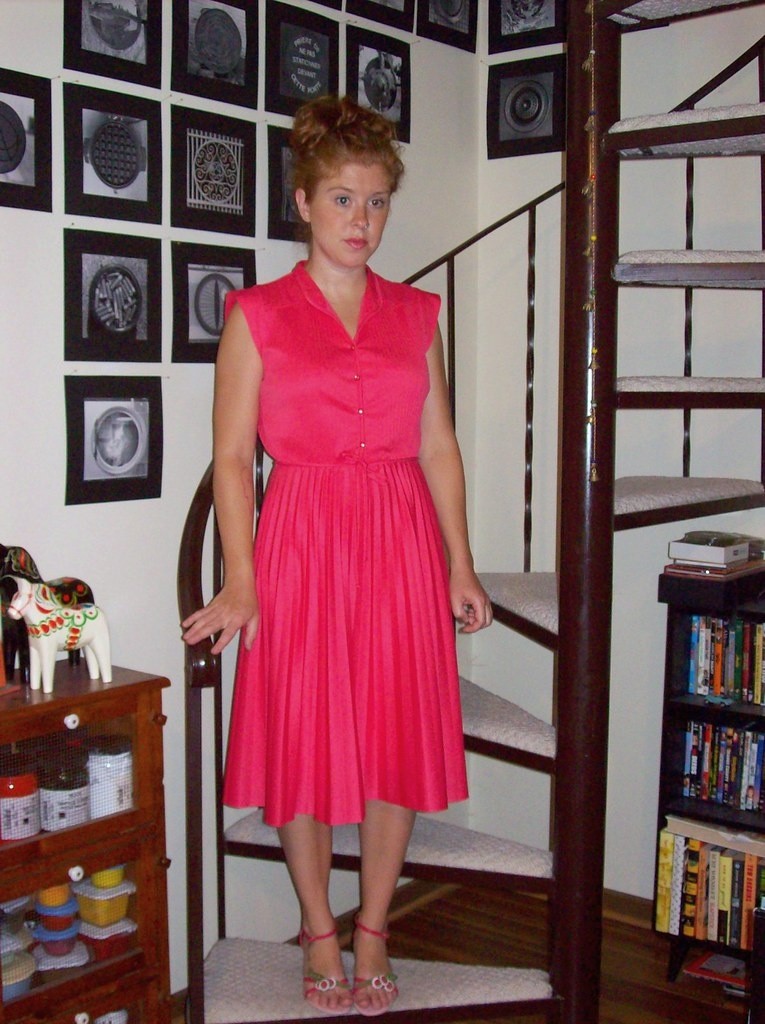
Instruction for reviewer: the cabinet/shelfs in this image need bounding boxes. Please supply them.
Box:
[0,653,169,1024]
[649,600,765,1000]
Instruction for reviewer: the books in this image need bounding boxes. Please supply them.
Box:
[683,721,765,810]
[654,829,765,988]
[688,616,765,706]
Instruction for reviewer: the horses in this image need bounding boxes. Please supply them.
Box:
[0,575,113,693]
[0,543,95,686]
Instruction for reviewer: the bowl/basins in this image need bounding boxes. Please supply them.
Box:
[0,863,136,1001]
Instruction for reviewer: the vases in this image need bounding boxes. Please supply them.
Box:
[0,0,566,507]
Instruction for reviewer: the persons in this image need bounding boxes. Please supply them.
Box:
[180,96,493,1015]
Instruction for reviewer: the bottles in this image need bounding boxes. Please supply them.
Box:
[0,730,132,844]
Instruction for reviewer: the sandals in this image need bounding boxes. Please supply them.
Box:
[351,910,399,1017]
[298,918,354,1014]
